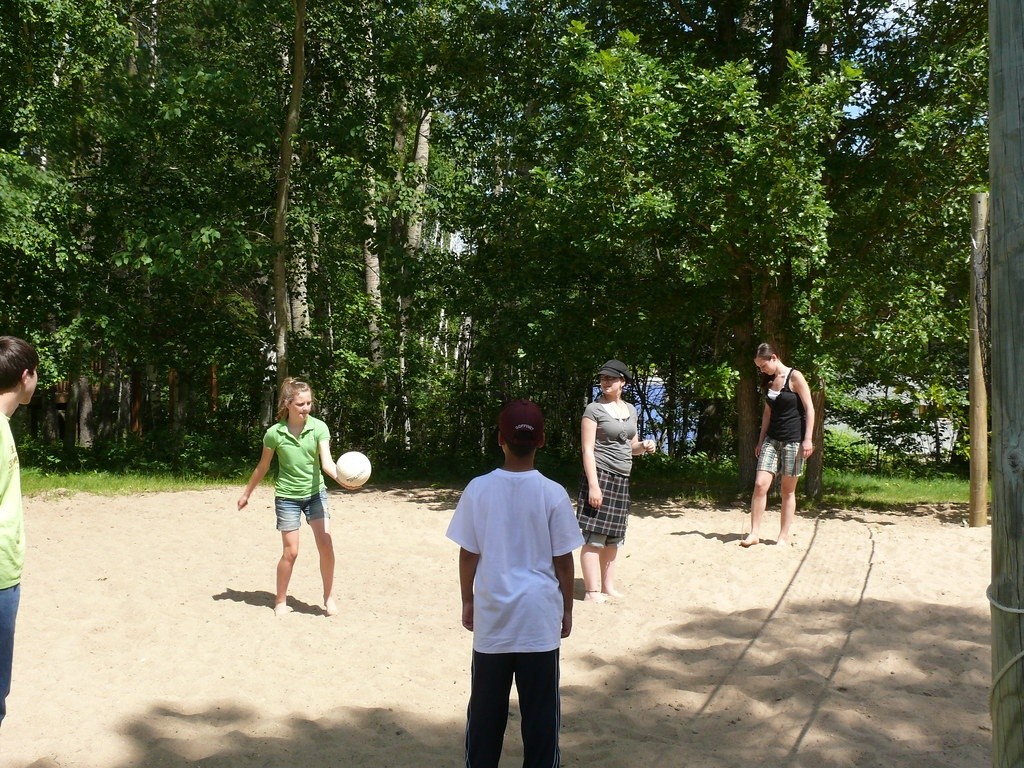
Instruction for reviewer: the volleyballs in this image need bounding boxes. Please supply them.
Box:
[336,451,373,491]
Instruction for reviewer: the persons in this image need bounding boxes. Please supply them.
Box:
[238,377,361,614]
[0,337,39,725]
[575,359,657,600]
[446,402,585,768]
[740,343,816,547]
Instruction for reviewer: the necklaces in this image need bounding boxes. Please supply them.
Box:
[606,399,623,422]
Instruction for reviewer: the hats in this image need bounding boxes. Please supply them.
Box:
[499,399,544,446]
[597,360,632,380]
